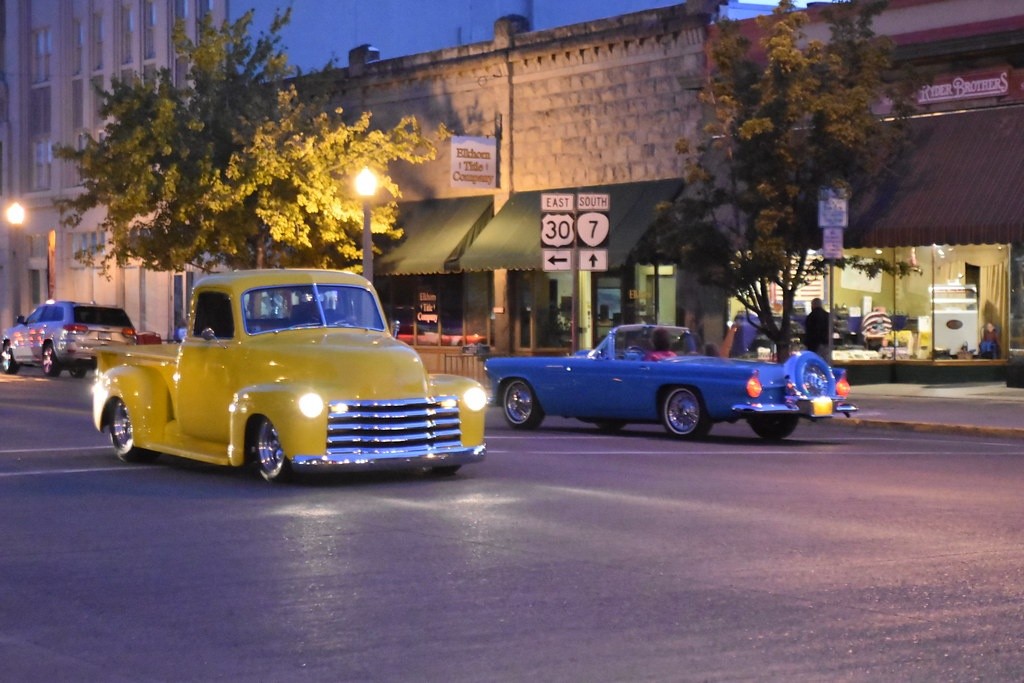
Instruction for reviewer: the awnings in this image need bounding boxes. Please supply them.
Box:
[690,105,1024,253]
[339,177,684,278]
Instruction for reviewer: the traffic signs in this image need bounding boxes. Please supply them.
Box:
[577,195,611,273]
[537,192,575,272]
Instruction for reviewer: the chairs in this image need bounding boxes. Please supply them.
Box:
[681,333,699,355]
[291,304,308,321]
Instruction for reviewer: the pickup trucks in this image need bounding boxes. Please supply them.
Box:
[91,269,489,486]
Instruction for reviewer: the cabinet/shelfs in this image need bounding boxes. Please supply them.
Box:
[926,283,979,358]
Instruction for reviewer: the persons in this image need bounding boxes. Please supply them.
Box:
[642,331,679,361]
[802,297,830,363]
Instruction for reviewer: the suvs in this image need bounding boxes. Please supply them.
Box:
[0,298,138,378]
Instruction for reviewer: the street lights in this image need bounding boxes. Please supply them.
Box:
[353,165,380,328]
[5,201,27,329]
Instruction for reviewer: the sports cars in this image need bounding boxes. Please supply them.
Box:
[482,322,862,445]
